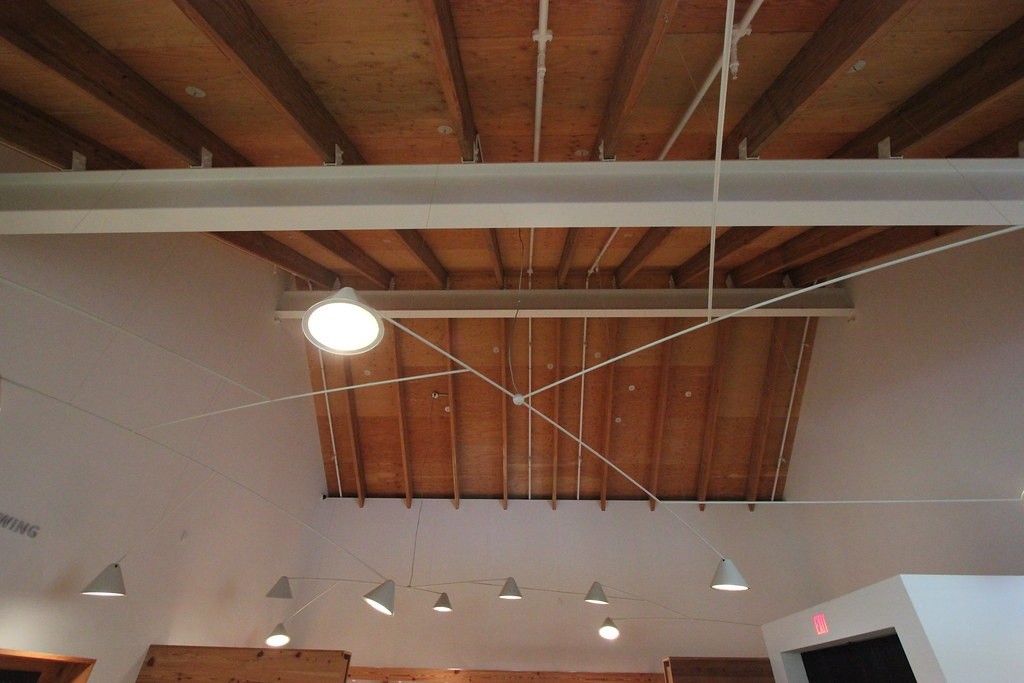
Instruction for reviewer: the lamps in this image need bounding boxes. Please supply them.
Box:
[80,563,126,597]
[432,593,452,613]
[711,557,750,592]
[266,576,294,600]
[598,617,620,640]
[265,623,291,647]
[361,580,395,617]
[498,577,522,600]
[300,286,385,355]
[583,582,609,605]
